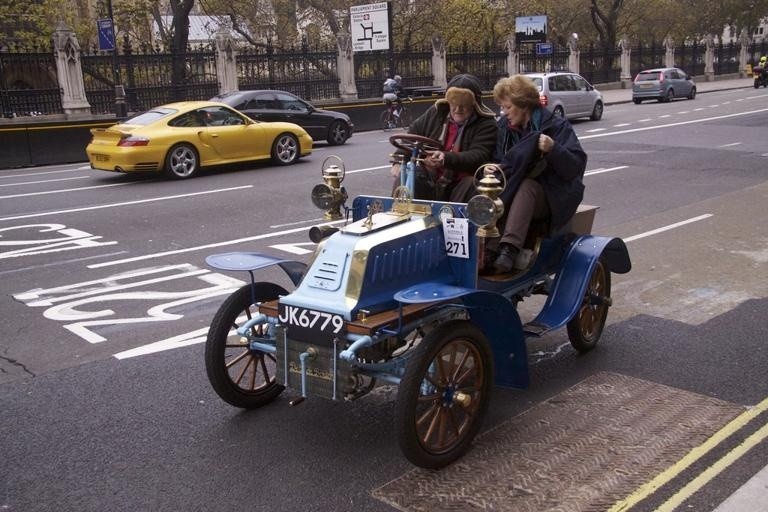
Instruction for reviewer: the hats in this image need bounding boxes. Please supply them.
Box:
[434,73,497,117]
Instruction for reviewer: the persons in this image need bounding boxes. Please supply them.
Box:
[758,56,768,88]
[477,73,587,274]
[382,74,414,129]
[389,73,498,204]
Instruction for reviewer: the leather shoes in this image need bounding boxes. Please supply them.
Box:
[486,242,520,274]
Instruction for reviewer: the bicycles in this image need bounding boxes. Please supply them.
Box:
[380,97,414,132]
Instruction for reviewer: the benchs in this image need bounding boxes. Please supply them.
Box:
[526,203,601,249]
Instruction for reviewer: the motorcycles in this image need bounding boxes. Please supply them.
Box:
[752,66,768,89]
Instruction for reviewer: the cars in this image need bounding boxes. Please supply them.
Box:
[522,72,603,121]
[207,90,355,146]
[633,67,696,104]
[84,100,313,179]
[206,133,632,471]
